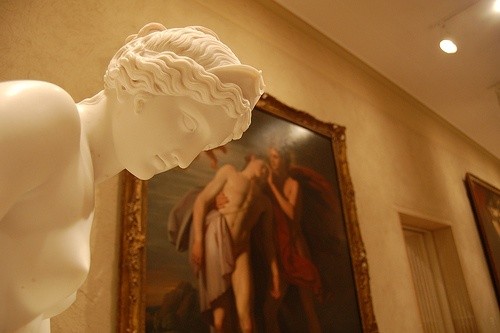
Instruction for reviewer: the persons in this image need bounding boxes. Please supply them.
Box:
[0,23,268,333]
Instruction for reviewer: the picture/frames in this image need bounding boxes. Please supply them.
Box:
[466,173,500,303]
[119,92,381,333]
